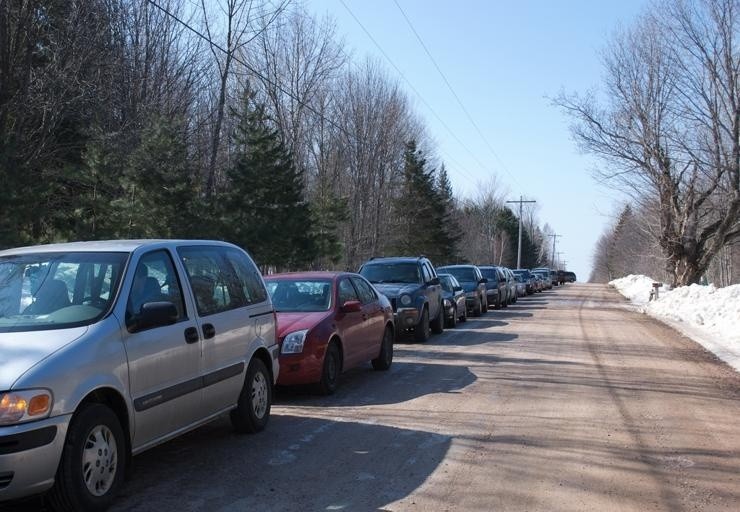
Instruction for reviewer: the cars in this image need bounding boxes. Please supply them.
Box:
[262,273,398,393]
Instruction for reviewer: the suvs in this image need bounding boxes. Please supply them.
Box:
[354,254,445,343]
[433,265,577,327]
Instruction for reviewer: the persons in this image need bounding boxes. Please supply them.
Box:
[117,262,156,333]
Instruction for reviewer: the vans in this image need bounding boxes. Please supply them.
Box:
[1,238,277,503]
